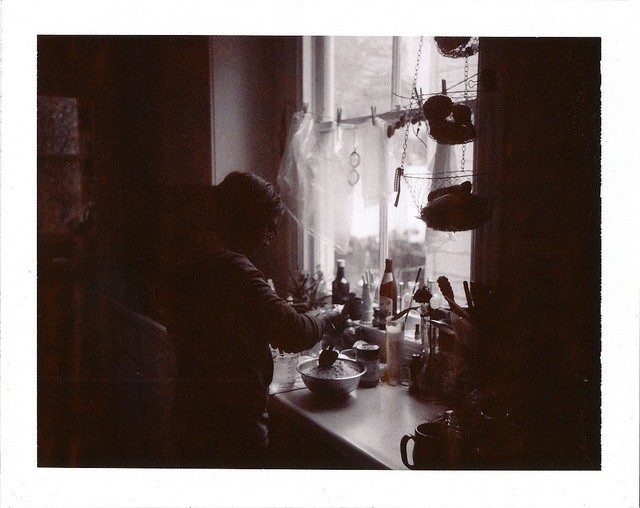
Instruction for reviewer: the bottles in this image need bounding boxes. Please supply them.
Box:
[378,257,398,330]
[332,259,350,304]
[402,280,421,312]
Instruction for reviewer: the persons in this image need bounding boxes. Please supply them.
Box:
[170,171,350,468]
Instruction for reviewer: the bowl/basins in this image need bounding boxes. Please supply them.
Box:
[296,356,367,397]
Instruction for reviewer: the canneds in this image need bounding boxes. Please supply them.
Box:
[356,344,381,386]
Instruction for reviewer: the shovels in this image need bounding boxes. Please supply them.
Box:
[318,302,350,367]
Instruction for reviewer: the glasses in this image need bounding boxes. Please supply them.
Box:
[268,228,277,240]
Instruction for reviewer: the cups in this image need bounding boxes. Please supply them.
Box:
[399,421,454,470]
